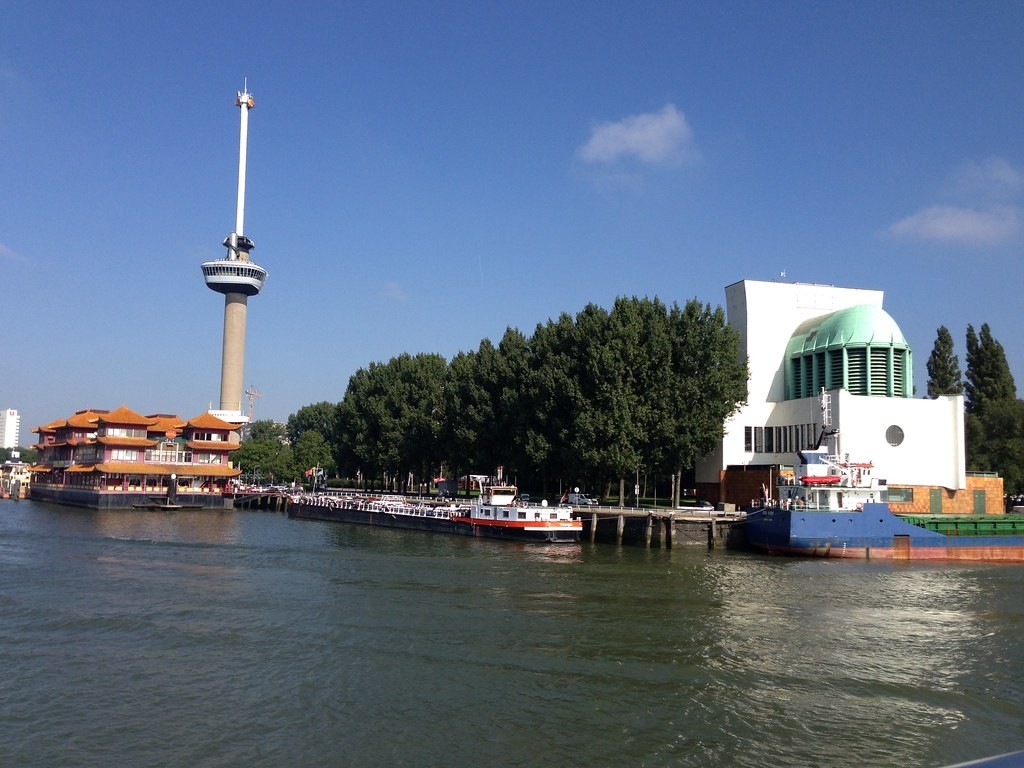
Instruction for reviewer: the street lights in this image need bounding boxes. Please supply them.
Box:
[170,474,177,493]
[574,487,580,506]
[252,464,261,484]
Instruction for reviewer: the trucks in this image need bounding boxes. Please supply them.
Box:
[438,480,458,501]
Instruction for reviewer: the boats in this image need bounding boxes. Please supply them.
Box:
[286,464,584,546]
[727,385,1024,565]
[130,496,204,511]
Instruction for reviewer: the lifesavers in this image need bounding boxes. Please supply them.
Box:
[856,502,863,510]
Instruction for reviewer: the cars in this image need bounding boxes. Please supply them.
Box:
[230,479,304,494]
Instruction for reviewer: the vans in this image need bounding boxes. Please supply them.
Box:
[568,494,599,506]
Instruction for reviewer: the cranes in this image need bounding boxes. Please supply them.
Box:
[245,385,263,421]
